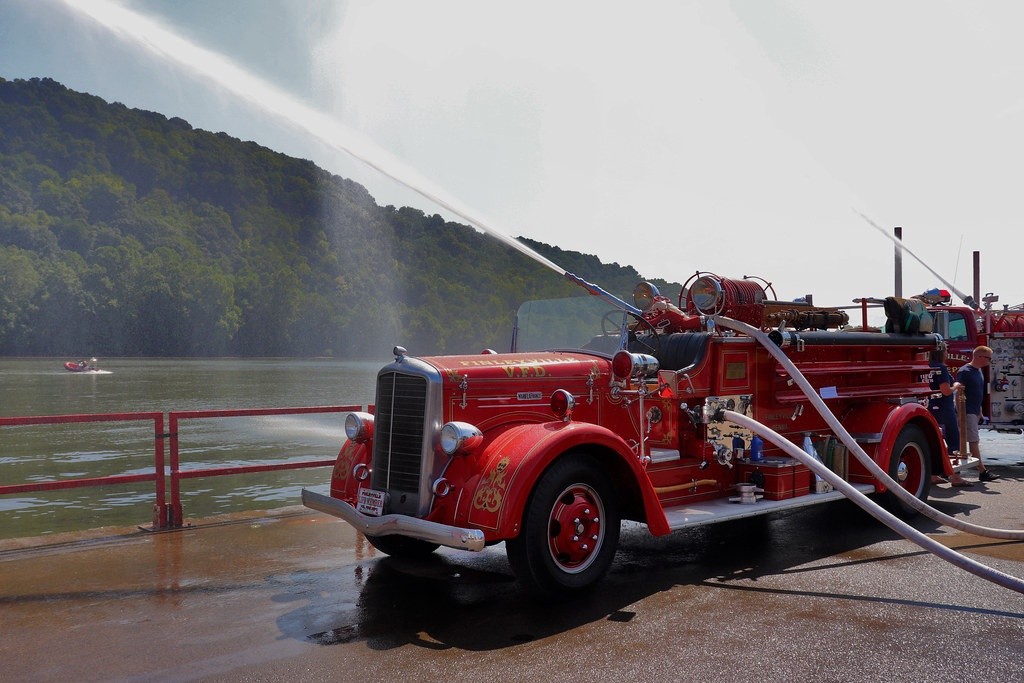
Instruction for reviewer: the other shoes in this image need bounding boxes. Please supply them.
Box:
[951,480,974,486]
[931,476,948,483]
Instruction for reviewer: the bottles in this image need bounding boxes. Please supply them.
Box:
[732,432,745,451]
[750,434,764,460]
[803,431,815,457]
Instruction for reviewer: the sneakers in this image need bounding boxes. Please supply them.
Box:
[978,470,1001,480]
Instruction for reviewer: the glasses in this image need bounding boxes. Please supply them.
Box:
[981,356,992,362]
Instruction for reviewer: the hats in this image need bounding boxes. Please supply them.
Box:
[939,342,948,351]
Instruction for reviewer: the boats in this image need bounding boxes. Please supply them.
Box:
[64,362,99,373]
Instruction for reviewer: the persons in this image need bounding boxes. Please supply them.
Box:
[931,342,975,486]
[956,345,1001,481]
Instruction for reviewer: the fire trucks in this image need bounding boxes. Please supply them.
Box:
[920,289,1024,437]
[293,269,981,611]
